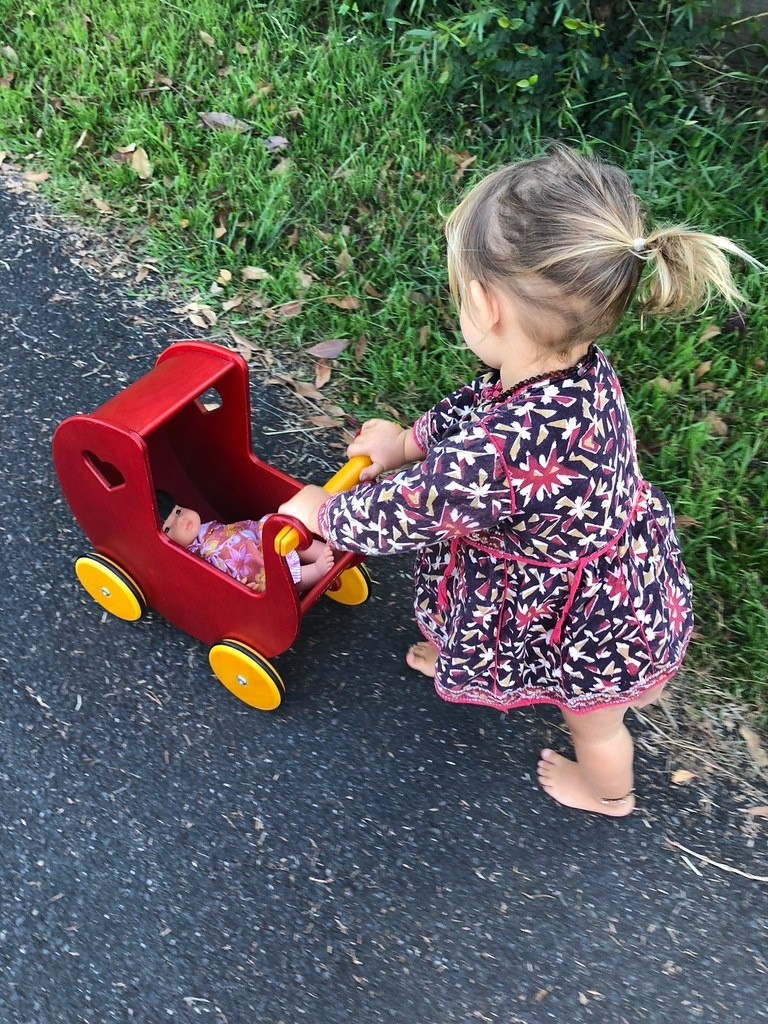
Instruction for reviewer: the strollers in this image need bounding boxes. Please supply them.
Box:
[52,342,374,711]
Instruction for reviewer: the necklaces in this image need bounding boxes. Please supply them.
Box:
[492,346,598,403]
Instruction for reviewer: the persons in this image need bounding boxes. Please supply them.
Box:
[278,148,766,818]
[157,496,335,593]
